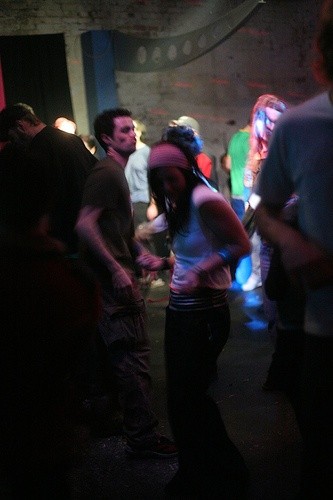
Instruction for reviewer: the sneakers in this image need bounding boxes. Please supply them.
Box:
[126,433,182,456]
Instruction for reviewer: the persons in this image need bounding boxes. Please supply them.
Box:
[0,103,99,451]
[124,116,201,289]
[73,108,179,456]
[253,1,333,500]
[136,144,253,500]
[217,94,298,348]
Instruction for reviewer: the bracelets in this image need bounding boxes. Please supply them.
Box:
[160,257,171,271]
[192,265,208,281]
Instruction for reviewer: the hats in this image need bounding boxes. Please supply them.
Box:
[149,143,191,170]
